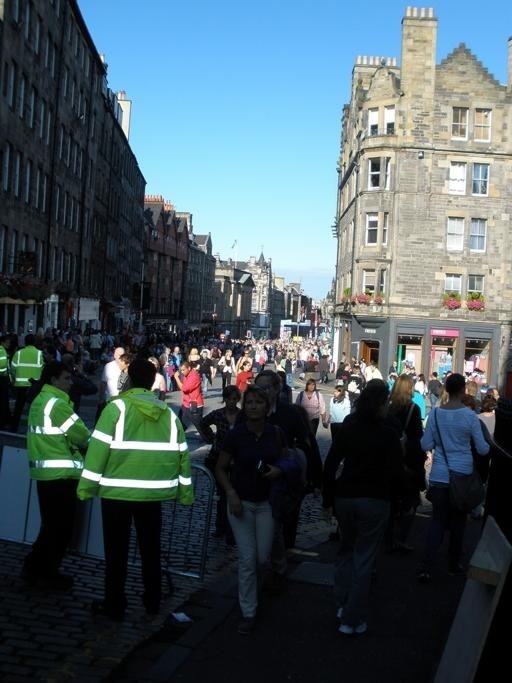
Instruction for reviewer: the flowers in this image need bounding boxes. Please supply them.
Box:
[442,291,485,310]
[341,287,385,305]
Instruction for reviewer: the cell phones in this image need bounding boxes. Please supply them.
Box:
[257,460,270,474]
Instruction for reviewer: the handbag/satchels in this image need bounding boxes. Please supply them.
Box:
[399,432,408,472]
[447,470,479,506]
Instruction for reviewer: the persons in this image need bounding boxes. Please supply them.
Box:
[252,335,383,389]
[19,361,91,588]
[201,362,500,635]
[77,360,195,619]
[2,327,253,444]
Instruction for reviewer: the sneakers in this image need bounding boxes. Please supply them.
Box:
[238,617,252,634]
[339,621,367,633]
[19,564,160,622]
